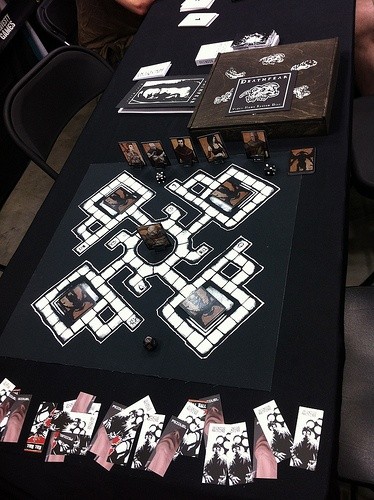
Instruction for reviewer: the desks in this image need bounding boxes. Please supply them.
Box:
[0,0,355,500]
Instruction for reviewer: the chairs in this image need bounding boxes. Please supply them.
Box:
[36,0,79,47]
[2,44,115,185]
